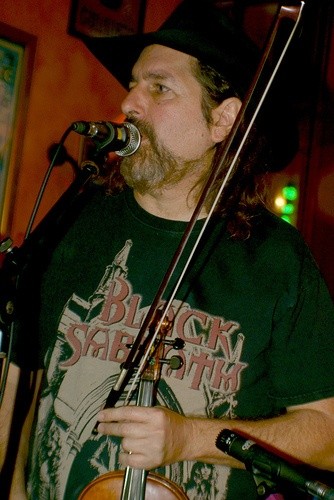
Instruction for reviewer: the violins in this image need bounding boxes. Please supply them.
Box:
[79,308,189,500]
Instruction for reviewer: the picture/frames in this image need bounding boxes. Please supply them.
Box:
[67,0,147,39]
[0,20,38,240]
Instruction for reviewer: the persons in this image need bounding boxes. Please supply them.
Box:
[0,28,334,500]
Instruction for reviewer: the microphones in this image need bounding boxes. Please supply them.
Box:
[215,428,334,500]
[73,120,141,157]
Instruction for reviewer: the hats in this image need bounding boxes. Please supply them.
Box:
[69,0,300,173]
